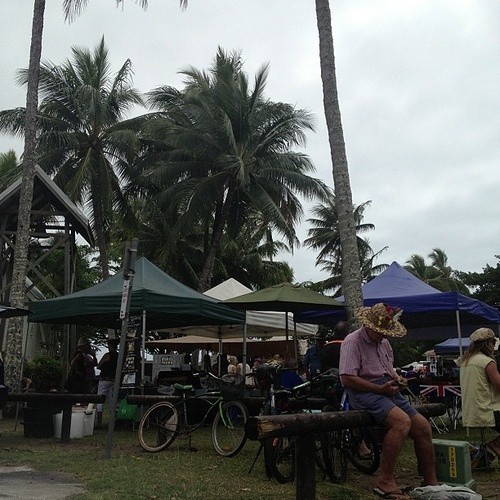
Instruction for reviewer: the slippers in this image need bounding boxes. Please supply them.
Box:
[373,486,410,500]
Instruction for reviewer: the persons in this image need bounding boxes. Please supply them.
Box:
[340,303,439,498]
[180,352,297,395]
[456,327,500,460]
[65,335,98,406]
[301,330,335,399]
[344,381,373,460]
[393,364,443,382]
[96,336,121,428]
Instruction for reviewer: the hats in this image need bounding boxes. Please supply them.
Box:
[77,336,90,346]
[313,331,326,339]
[354,302,407,338]
[470,328,498,342]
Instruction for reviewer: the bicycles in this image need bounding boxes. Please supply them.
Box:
[248,379,338,484]
[309,365,379,483]
[139,370,249,457]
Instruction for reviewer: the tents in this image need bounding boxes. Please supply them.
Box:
[219,280,345,419]
[295,261,500,439]
[0,303,35,423]
[153,277,319,432]
[13,255,247,434]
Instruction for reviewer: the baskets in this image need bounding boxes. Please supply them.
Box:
[220,373,245,401]
